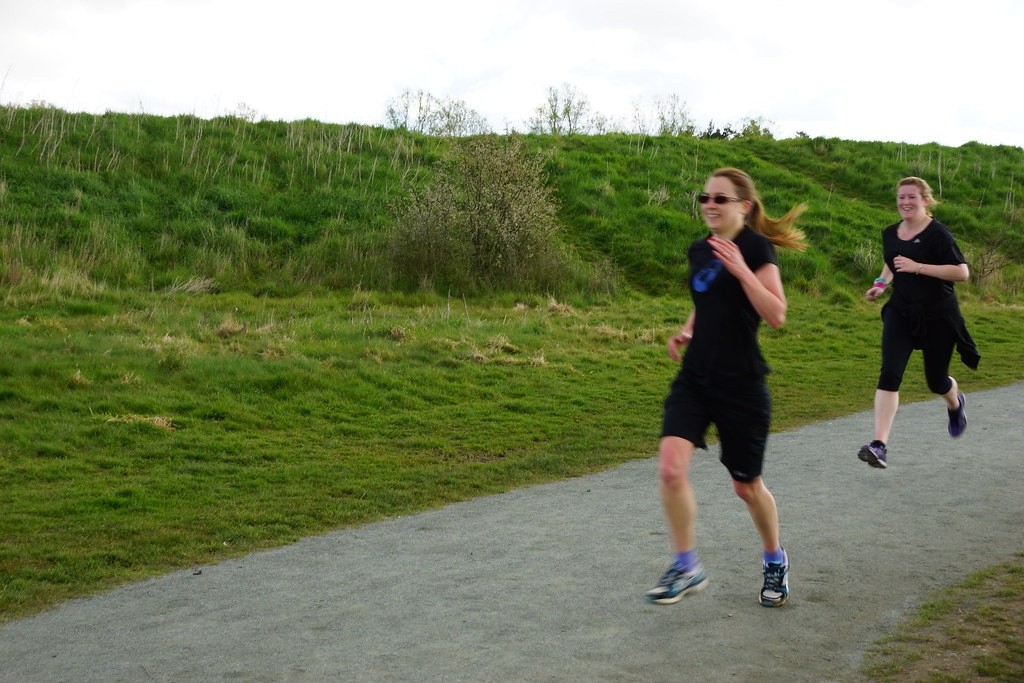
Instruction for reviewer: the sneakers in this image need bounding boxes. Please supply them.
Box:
[947,392,967,438]
[759,545,789,608]
[858,440,888,469]
[644,558,709,605]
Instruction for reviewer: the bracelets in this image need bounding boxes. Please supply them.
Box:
[873,277,888,285]
[872,282,886,289]
[681,331,692,339]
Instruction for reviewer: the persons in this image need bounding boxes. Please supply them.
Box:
[857,176,982,470]
[640,166,807,608]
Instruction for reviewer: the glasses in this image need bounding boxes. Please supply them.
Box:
[696,193,746,204]
